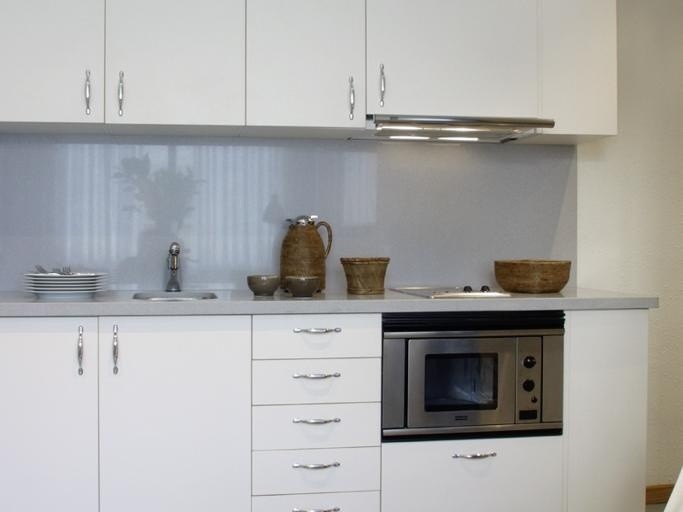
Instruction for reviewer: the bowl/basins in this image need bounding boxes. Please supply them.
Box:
[245,274,279,298]
[491,257,572,293]
[282,274,322,298]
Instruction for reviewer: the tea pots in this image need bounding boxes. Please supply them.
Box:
[280,211,333,292]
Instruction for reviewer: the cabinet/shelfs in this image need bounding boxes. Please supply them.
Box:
[246,0,366,142]
[0,0,246,136]
[564,307,650,512]
[0,314,252,512]
[381,435,564,512]
[249,314,380,512]
[362,0,617,143]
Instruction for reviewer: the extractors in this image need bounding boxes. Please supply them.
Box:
[372,113,556,149]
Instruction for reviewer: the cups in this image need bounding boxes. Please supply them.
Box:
[339,253,389,293]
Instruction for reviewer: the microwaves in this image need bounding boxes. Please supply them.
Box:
[382,329,563,437]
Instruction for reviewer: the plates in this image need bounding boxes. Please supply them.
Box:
[25,271,107,299]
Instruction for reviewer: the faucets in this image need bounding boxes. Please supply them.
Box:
[165,242,182,292]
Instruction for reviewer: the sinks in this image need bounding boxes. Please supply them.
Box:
[132,291,218,304]
